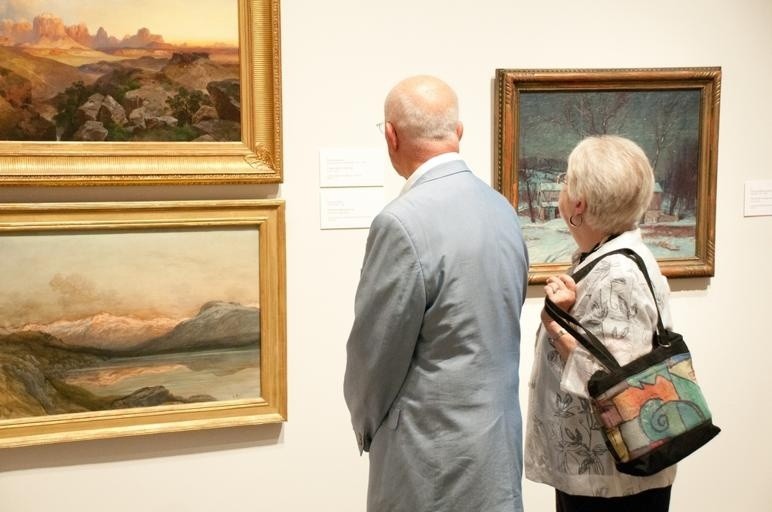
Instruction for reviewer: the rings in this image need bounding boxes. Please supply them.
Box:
[553,285,564,293]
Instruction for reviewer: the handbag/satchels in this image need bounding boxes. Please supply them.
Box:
[587,328,723,478]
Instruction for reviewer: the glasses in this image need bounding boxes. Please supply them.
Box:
[556,173,568,186]
[376,120,390,135]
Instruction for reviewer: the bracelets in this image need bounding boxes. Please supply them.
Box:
[546,328,570,348]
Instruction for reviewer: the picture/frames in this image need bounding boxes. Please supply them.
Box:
[0,200,287,450]
[0,0,283,186]
[494,67,722,286]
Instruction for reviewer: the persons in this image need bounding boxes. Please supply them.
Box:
[520,133,686,511]
[342,71,530,512]
